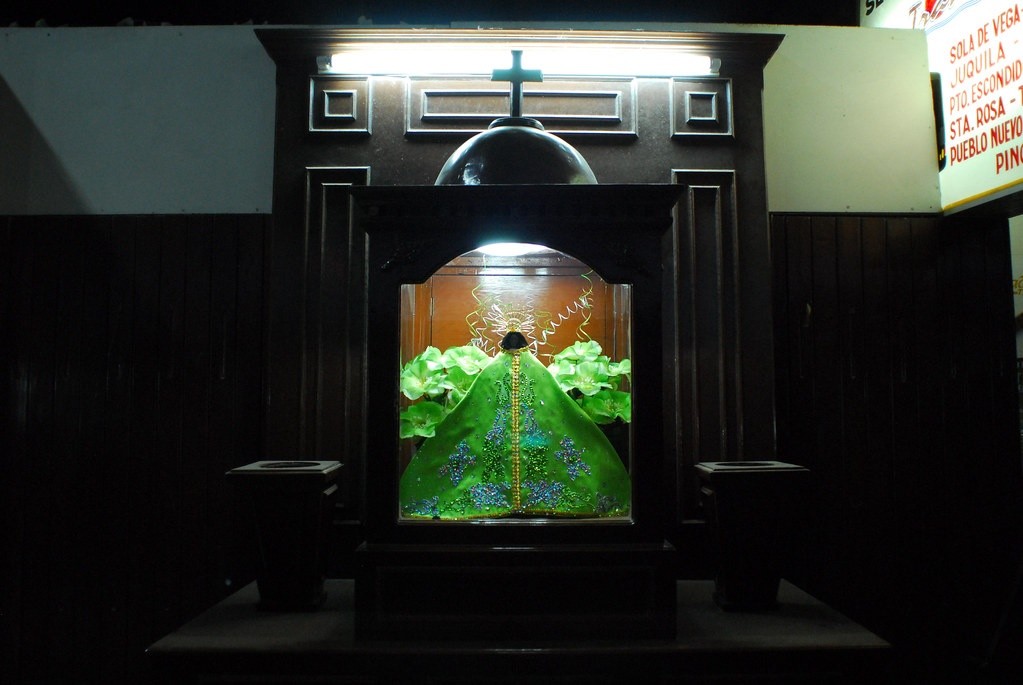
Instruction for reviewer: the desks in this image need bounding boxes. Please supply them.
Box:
[149,575,893,685]
